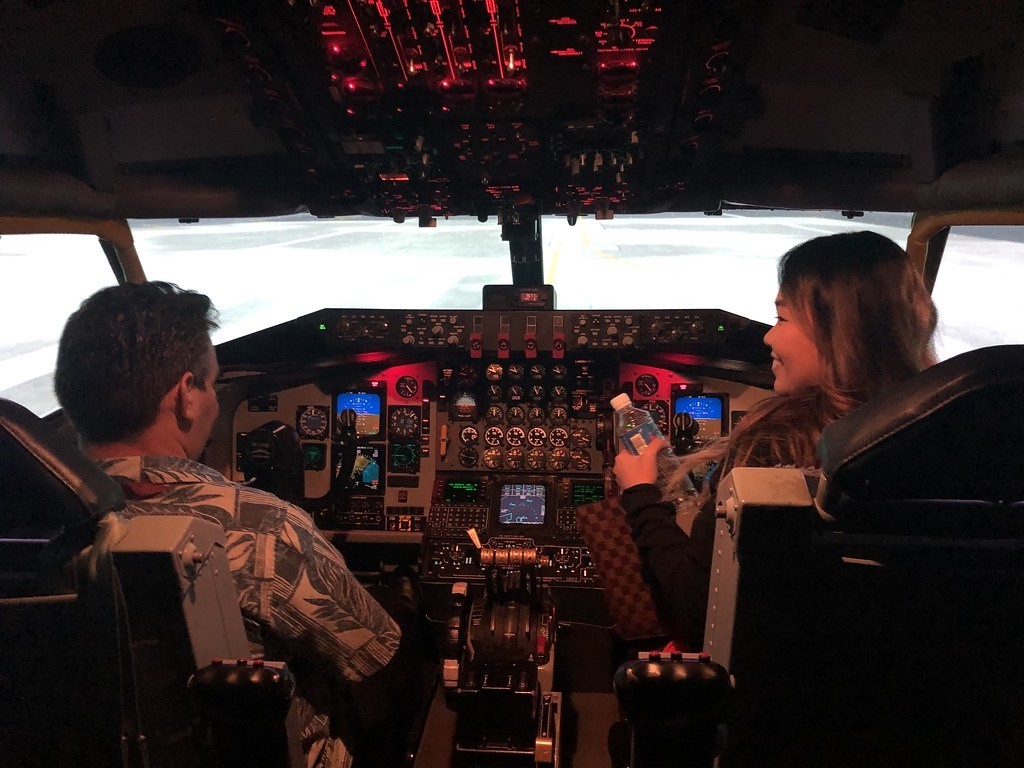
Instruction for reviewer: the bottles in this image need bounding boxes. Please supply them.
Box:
[609,392,701,517]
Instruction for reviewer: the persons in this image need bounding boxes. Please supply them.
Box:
[54,280,402,768]
[611,230,937,654]
